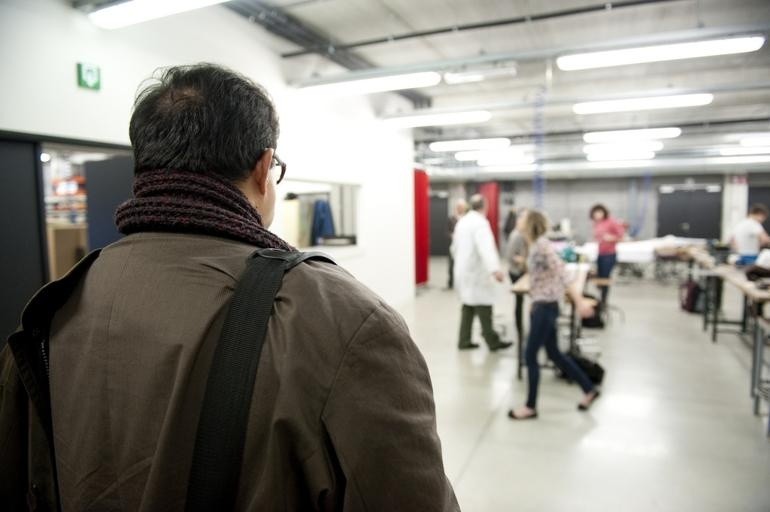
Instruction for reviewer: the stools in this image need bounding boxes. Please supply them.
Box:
[589,277,626,327]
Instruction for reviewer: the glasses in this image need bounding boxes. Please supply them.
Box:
[263,147,288,185]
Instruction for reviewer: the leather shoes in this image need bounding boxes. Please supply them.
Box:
[509,409,537,419]
[489,341,513,351]
[578,392,599,410]
[459,343,479,349]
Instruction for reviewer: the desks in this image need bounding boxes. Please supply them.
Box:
[678,247,770,436]
[576,244,708,283]
[512,261,590,382]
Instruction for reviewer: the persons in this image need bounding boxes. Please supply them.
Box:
[590,206,627,310]
[449,193,513,353]
[0,62,465,511]
[505,208,530,338]
[503,199,518,241]
[731,204,770,265]
[509,210,600,421]
[444,199,465,288]
